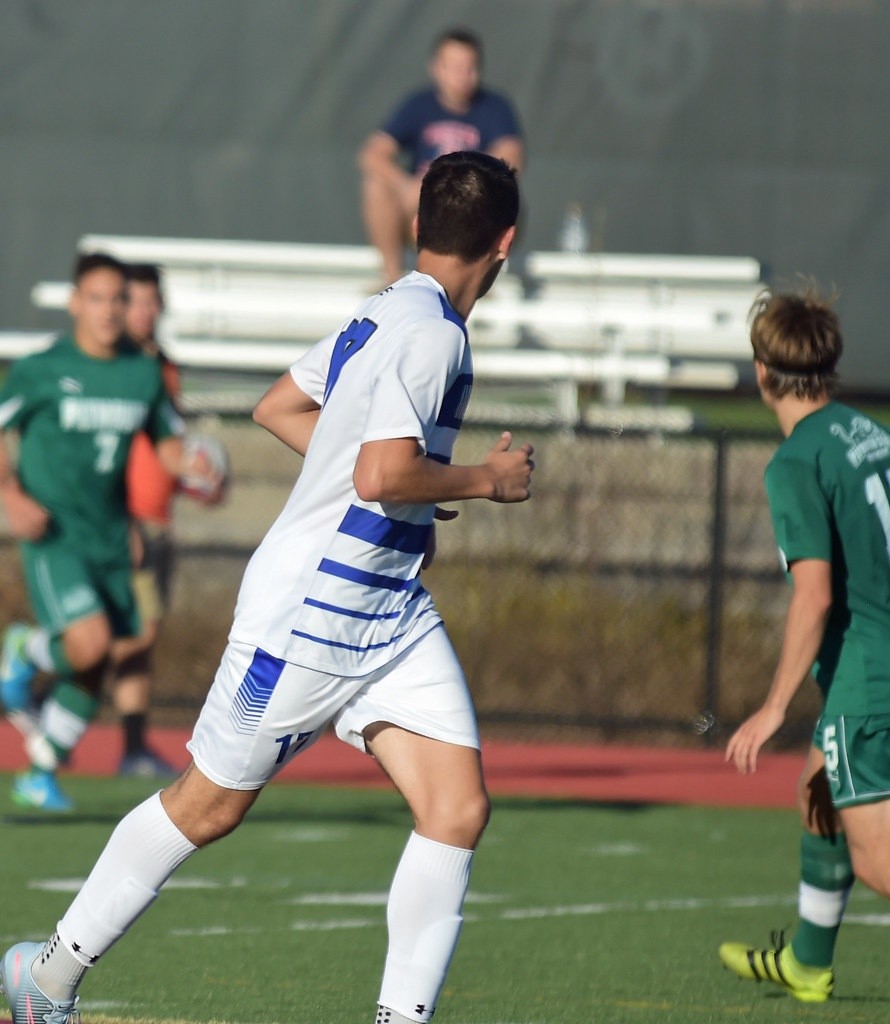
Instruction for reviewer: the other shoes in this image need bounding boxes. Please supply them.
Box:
[11,774,74,810]
[119,754,177,778]
[1,621,36,710]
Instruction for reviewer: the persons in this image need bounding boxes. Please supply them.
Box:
[359,28,523,297]
[717,274,890,1002]
[1,150,535,1023]
[2,252,230,815]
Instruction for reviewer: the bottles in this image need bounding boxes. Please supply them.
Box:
[559,204,591,258]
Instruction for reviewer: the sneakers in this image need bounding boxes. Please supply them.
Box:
[719,930,834,1003]
[2,942,80,1024]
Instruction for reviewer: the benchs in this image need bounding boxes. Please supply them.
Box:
[30,235,774,440]
